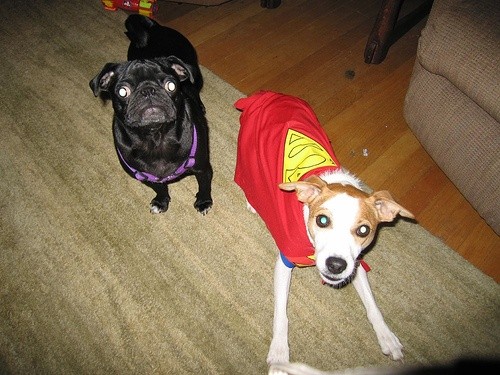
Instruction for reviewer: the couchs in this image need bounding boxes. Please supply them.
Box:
[403,0,500,238]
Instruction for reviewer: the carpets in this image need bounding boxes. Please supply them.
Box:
[1,0,499,374]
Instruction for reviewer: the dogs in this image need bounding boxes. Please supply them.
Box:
[233,90,416,367]
[89,13,214,218]
